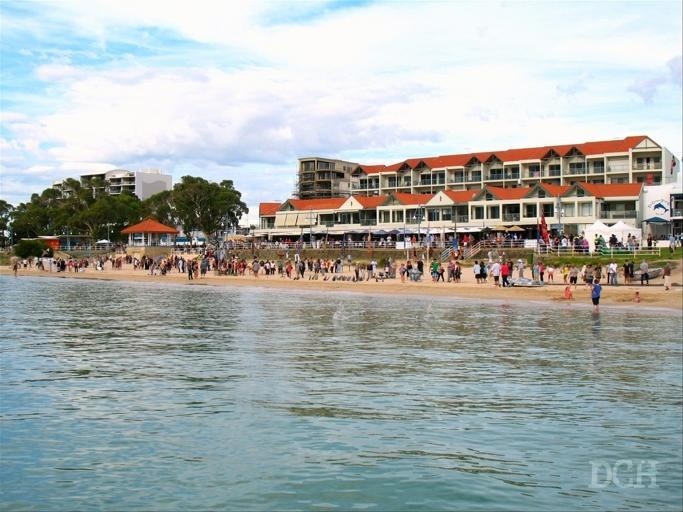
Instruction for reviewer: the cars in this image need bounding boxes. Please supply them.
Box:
[222,241,234,250]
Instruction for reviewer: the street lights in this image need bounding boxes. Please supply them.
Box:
[222,232,228,240]
[554,193,565,236]
[412,200,426,241]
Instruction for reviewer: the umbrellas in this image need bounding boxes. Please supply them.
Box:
[506,226,525,231]
[491,225,509,231]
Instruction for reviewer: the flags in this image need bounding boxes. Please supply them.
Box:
[541,209,550,246]
